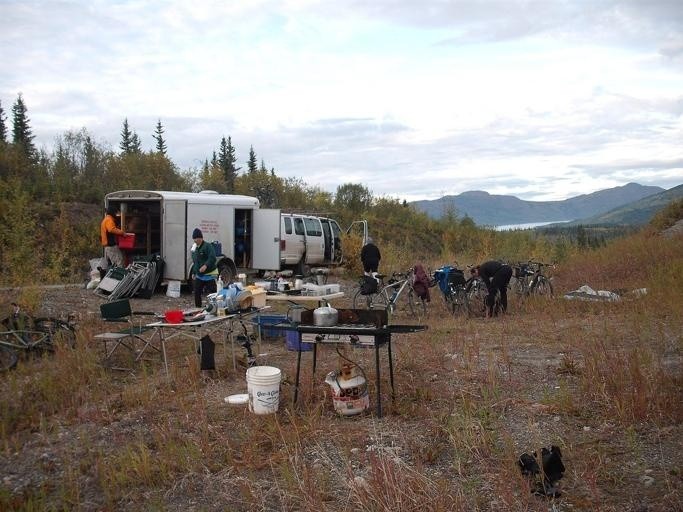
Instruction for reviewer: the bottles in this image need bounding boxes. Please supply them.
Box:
[529,280,533,287]
[296,275,302,290]
[293,305,301,322]
[216,278,223,297]
[389,290,397,301]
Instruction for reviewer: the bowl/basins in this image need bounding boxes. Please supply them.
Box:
[165,311,183,324]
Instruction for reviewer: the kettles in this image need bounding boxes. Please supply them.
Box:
[313,299,338,326]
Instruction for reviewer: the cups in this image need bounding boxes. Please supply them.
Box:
[316,274,324,285]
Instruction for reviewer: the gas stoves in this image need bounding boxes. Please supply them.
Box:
[296,320,378,346]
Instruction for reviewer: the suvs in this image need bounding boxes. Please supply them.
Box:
[256,211,369,276]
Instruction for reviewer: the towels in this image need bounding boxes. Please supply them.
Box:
[196,334,216,370]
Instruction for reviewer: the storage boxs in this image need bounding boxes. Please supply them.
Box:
[300,283,340,296]
[116,233,136,249]
[252,291,267,308]
[285,318,313,351]
[253,315,291,337]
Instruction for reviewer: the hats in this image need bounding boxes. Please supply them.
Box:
[192,228,203,239]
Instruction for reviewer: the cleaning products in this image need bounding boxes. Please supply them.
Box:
[216,276,223,292]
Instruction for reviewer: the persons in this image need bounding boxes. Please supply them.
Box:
[360,237,381,283]
[190,228,216,308]
[101,206,129,266]
[470,260,513,318]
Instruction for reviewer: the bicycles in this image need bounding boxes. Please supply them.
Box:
[0,300,79,374]
[351,257,557,322]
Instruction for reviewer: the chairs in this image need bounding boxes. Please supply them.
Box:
[99,298,161,371]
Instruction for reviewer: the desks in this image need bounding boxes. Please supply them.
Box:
[258,292,346,310]
[146,305,270,378]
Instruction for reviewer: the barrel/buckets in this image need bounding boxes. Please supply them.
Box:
[246,366,281,415]
